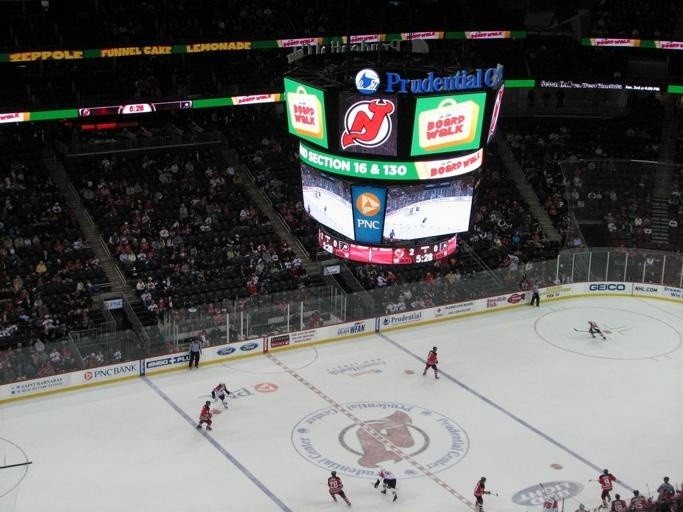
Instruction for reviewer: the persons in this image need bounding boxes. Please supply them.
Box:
[1,111,683,386]
[543,490,683,512]
[196,401,212,430]
[598,469,616,508]
[212,383,230,409]
[374,469,397,502]
[328,471,351,506]
[657,477,675,497]
[474,477,491,512]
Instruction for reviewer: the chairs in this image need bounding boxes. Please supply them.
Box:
[0,103,683,384]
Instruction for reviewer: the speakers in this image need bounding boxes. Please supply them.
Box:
[523,7,582,43]
